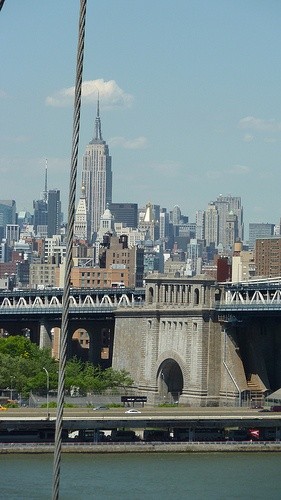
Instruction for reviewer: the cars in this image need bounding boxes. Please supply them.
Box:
[93,406,110,411]
[258,409,271,412]
[0,427,281,445]
[125,409,142,414]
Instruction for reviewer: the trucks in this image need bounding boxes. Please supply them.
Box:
[270,406,281,412]
